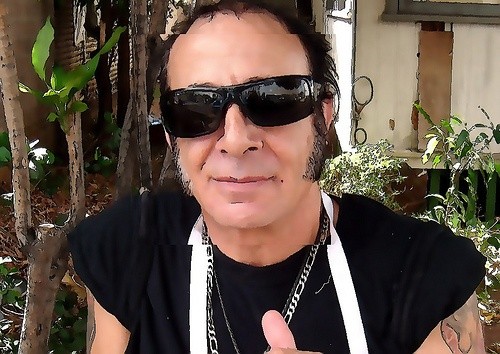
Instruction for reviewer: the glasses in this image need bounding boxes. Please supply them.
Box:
[159,75,322,138]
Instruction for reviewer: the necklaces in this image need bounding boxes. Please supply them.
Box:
[196,209,328,354]
[211,256,306,354]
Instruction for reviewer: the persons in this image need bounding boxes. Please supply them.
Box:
[66,0,488,353]
